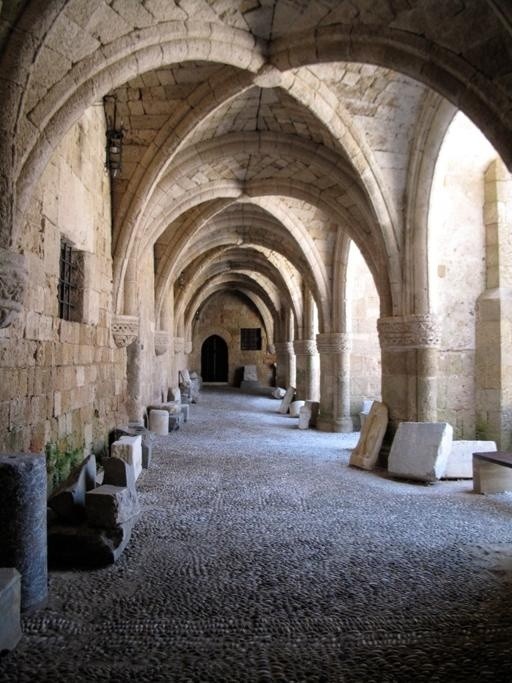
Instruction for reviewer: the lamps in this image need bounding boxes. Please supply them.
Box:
[102,95,126,182]
